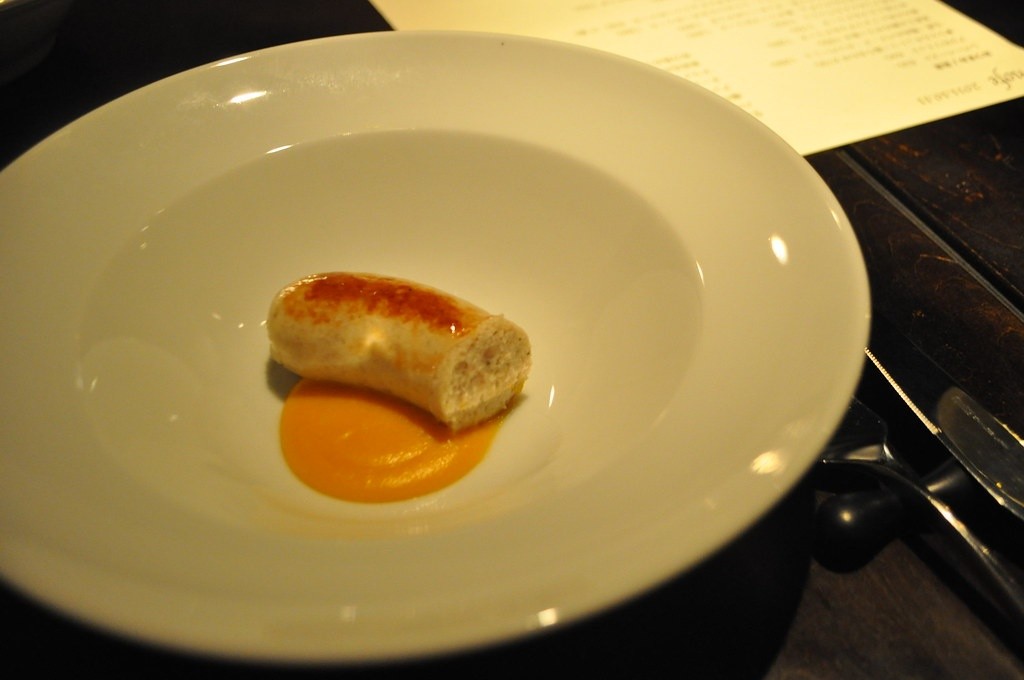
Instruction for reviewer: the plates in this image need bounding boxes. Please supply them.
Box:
[0,30,871,670]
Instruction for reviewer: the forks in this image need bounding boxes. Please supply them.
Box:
[817,395,1023,616]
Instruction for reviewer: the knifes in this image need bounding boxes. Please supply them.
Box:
[868,328,1024,521]
